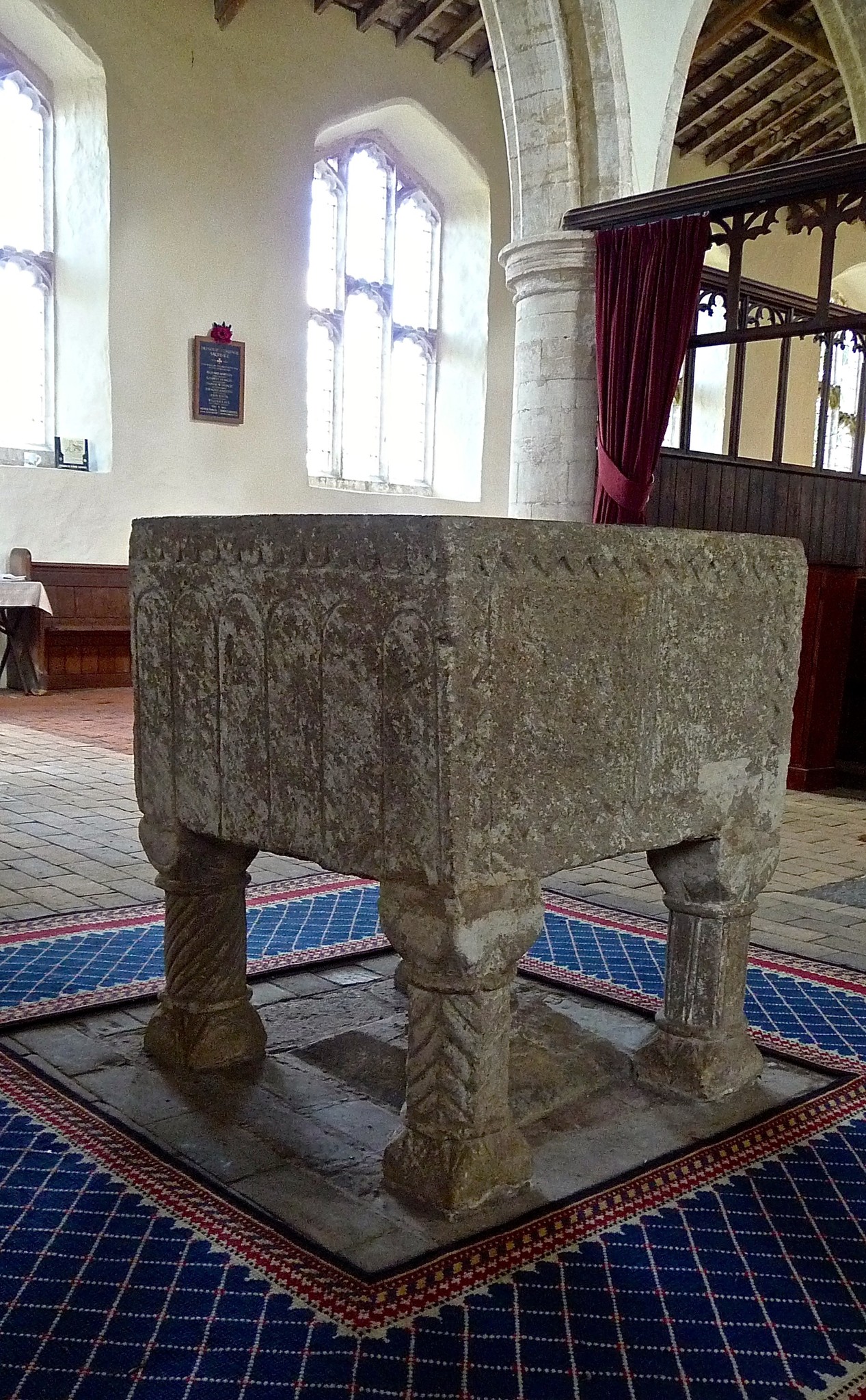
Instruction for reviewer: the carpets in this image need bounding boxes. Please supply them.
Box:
[0,875,865,1399]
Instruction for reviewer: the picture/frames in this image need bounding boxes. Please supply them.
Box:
[191,335,246,424]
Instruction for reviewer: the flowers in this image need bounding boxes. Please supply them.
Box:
[211,321,232,344]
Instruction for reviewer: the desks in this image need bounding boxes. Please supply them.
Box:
[0,581,53,696]
